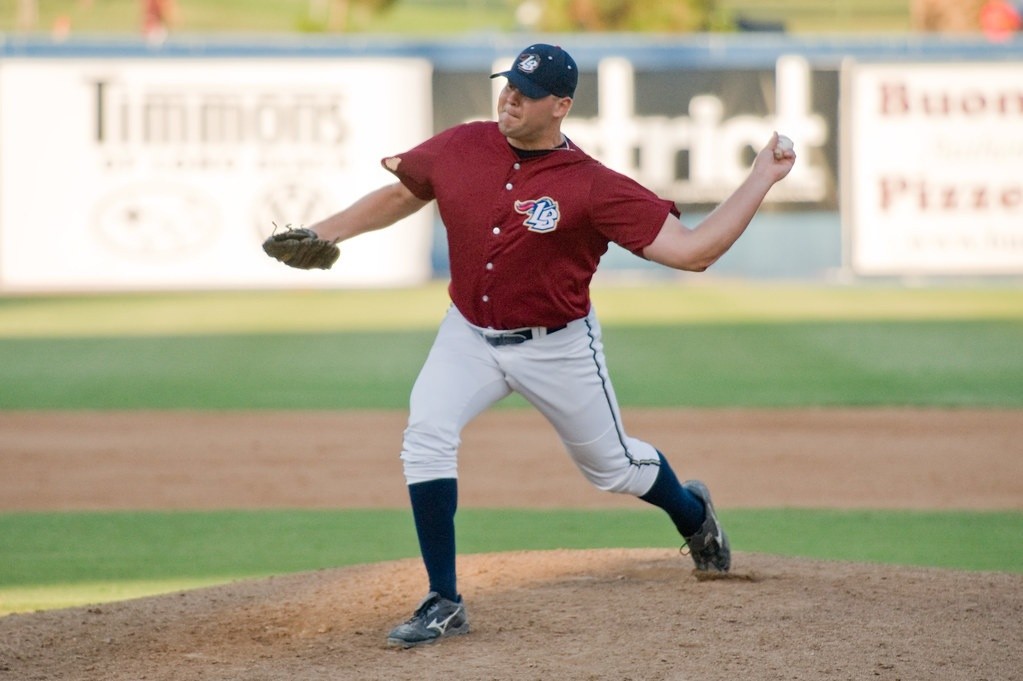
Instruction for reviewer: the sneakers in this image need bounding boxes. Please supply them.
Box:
[679,479,731,573]
[386,592,470,648]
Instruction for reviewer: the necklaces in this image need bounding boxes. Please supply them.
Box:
[549,135,569,151]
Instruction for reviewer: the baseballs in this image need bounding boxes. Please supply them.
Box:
[776,134,795,154]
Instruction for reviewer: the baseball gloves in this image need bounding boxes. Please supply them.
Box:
[262,221,342,271]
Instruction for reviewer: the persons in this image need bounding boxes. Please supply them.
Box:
[263,43,796,647]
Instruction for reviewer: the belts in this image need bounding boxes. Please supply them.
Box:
[480,323,567,347]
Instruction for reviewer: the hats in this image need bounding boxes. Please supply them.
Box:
[490,44,578,99]
